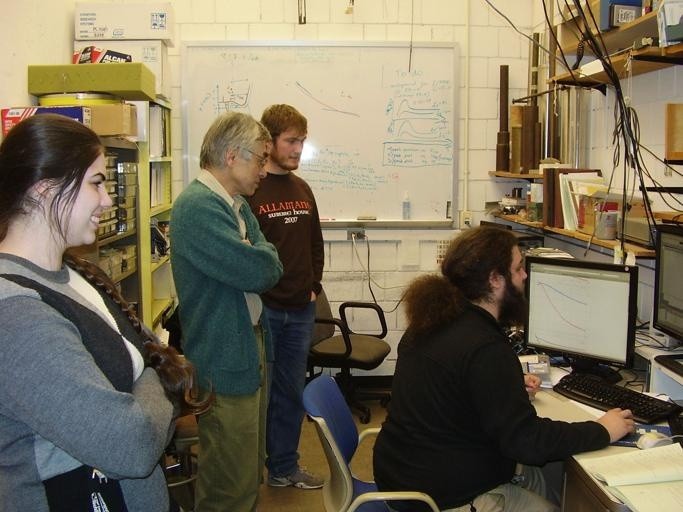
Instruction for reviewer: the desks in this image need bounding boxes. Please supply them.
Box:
[507,328,683,511]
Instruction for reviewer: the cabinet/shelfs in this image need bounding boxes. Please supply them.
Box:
[64,136,142,326]
[139,100,177,343]
[486,168,543,229]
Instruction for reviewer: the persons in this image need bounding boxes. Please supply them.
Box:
[244,104,326,489]
[373,226,636,512]
[0,112,216,512]
[169,112,284,512]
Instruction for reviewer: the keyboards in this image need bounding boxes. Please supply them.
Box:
[552,369,682,425]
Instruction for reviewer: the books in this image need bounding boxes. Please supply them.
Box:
[577,442,683,512]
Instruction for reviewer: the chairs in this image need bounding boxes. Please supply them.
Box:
[305,282,393,425]
[300,373,447,512]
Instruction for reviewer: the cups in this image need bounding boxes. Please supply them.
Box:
[595,210,617,240]
[511,188,521,198]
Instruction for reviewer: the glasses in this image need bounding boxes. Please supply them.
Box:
[243,146,270,168]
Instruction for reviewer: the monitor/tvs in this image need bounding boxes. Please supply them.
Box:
[653,225,683,375]
[525,257,636,384]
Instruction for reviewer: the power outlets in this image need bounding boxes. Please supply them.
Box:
[345,228,366,241]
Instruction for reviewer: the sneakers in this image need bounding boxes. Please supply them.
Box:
[266,466,325,490]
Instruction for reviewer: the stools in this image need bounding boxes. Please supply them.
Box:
[163,406,199,510]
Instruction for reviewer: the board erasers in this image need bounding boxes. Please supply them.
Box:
[357,216,377,220]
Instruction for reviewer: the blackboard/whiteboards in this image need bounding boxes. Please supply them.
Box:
[180,40,459,229]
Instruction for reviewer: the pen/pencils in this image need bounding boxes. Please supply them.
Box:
[645,358,651,392]
[320,219,335,220]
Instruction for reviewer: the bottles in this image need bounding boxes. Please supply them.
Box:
[402,192,409,220]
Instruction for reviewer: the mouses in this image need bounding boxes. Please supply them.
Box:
[637,431,672,449]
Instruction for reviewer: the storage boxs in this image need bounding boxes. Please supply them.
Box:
[72,3,181,49]
[71,41,169,99]
